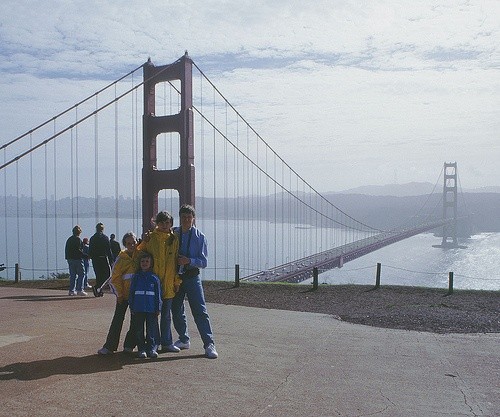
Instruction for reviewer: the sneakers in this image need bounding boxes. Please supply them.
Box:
[148,350,158,358]
[84,285,92,289]
[137,352,147,358]
[205,343,218,358]
[77,291,87,296]
[69,291,77,295]
[93,286,98,296]
[174,340,190,348]
[99,292,103,296]
[154,344,159,351]
[124,348,132,352]
[162,345,180,352]
[98,346,113,354]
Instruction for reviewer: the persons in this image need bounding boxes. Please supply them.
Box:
[97,206,219,358]
[108,233,119,270]
[88,223,115,296]
[65,225,87,296]
[82,238,93,288]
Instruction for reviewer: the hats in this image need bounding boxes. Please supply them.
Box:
[83,238,88,241]
